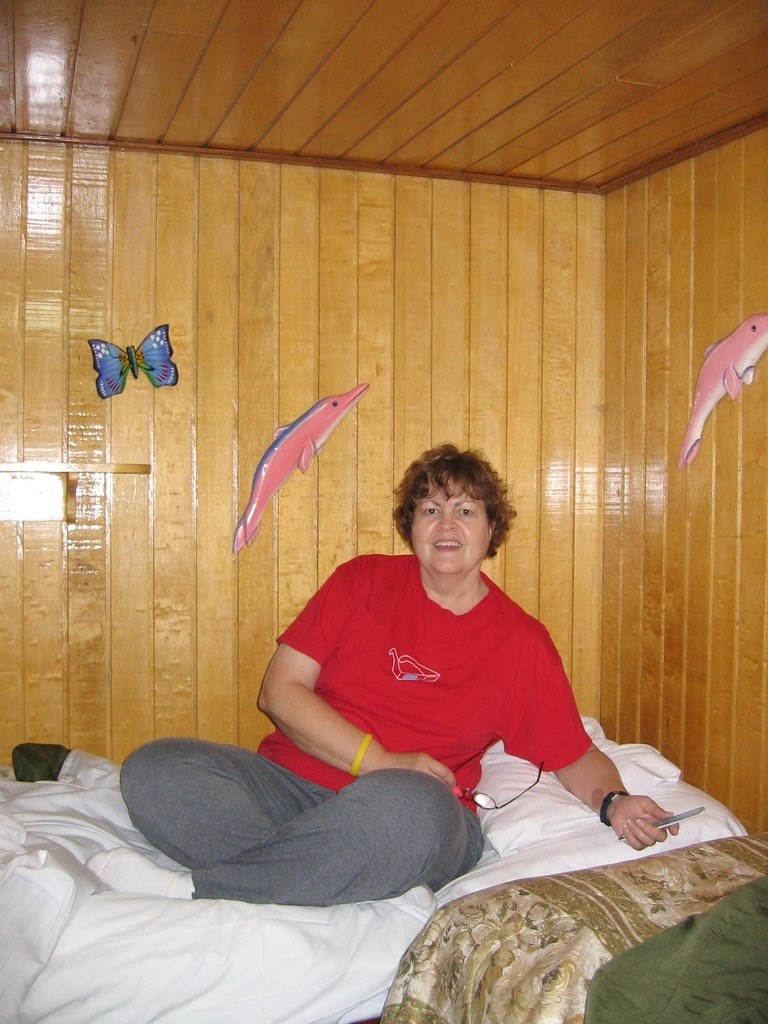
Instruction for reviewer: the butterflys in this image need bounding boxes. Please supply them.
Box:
[86,323,181,399]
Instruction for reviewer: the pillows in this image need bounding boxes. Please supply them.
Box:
[471,717,684,857]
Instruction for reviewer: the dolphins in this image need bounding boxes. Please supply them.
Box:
[675,310,768,472]
[229,380,373,555]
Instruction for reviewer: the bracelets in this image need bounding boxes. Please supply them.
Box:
[599,789,632,827]
[350,732,373,777]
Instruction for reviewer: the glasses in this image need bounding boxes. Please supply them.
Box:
[453,737,544,810]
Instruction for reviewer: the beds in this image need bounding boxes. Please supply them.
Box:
[1,712,756,1022]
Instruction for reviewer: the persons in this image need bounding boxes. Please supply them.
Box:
[120,443,678,908]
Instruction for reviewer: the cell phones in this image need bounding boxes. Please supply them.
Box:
[619,807,705,841]
